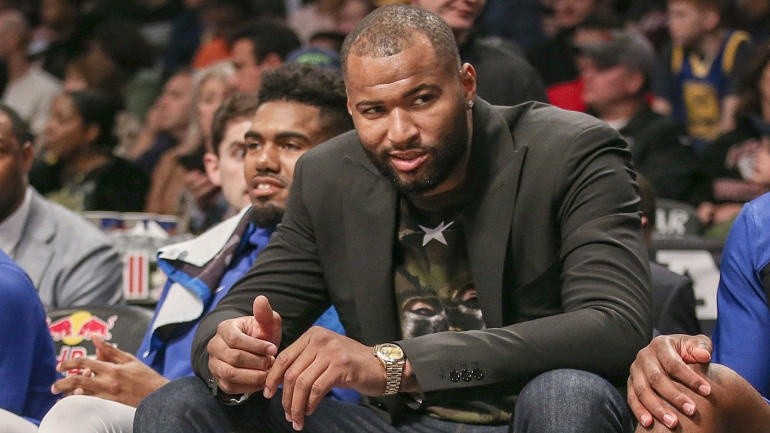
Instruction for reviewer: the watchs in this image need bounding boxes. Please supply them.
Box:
[372,342,405,397]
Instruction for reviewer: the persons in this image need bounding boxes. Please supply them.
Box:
[0,65,354,432]
[627,190,770,433]
[0,102,126,312]
[133,6,652,433]
[0,249,59,432]
[204,92,258,215]
[1,0,770,229]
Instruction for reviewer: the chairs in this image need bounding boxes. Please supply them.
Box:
[45,201,724,401]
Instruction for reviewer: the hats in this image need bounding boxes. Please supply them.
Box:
[569,17,656,77]
